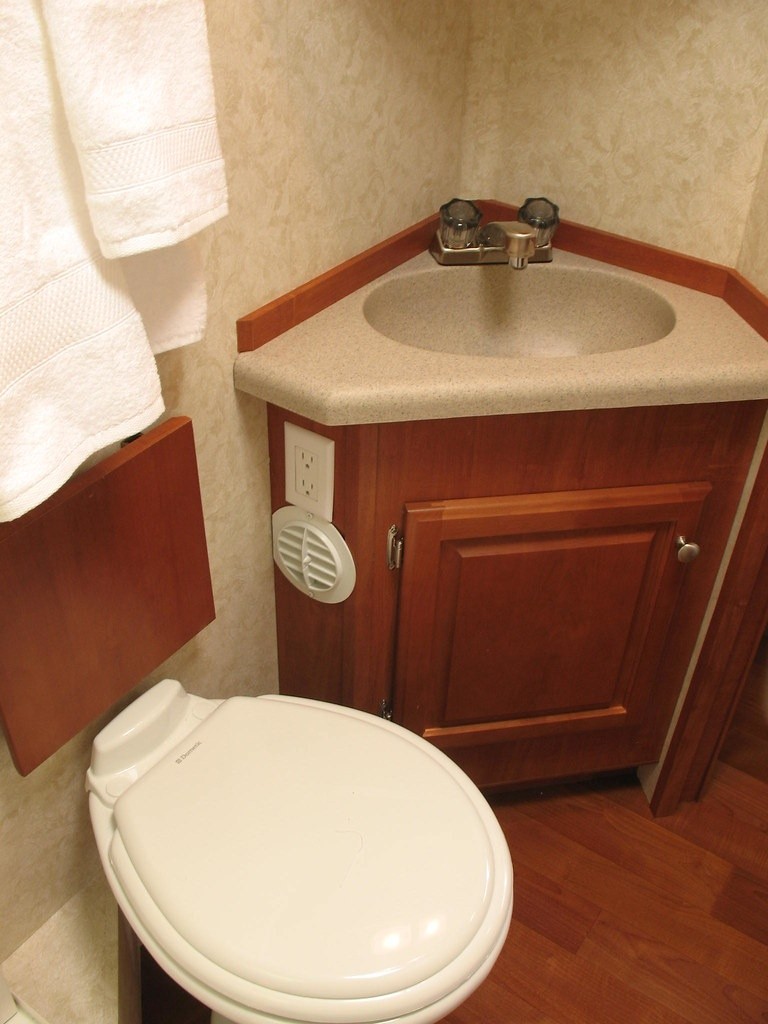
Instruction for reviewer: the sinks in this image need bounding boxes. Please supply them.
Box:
[361,263,677,360]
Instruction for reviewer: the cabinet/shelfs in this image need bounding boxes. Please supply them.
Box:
[265,397,768,790]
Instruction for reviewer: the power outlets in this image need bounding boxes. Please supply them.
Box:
[283,420,335,525]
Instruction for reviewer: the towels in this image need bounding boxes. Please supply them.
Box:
[0,1,231,527]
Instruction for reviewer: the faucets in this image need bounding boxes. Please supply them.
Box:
[428,196,561,272]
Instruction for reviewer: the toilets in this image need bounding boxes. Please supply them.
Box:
[83,676,516,1024]
